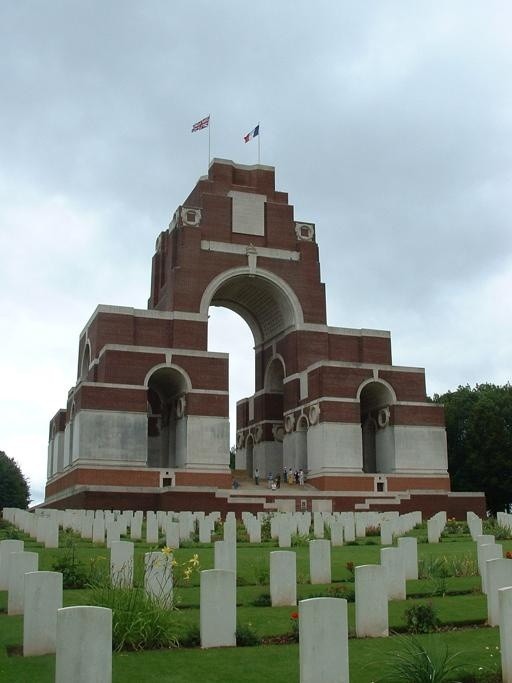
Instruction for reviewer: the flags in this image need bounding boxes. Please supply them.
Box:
[243,124,259,143]
[190,116,210,132]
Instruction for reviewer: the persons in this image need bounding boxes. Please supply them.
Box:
[231,465,307,490]
